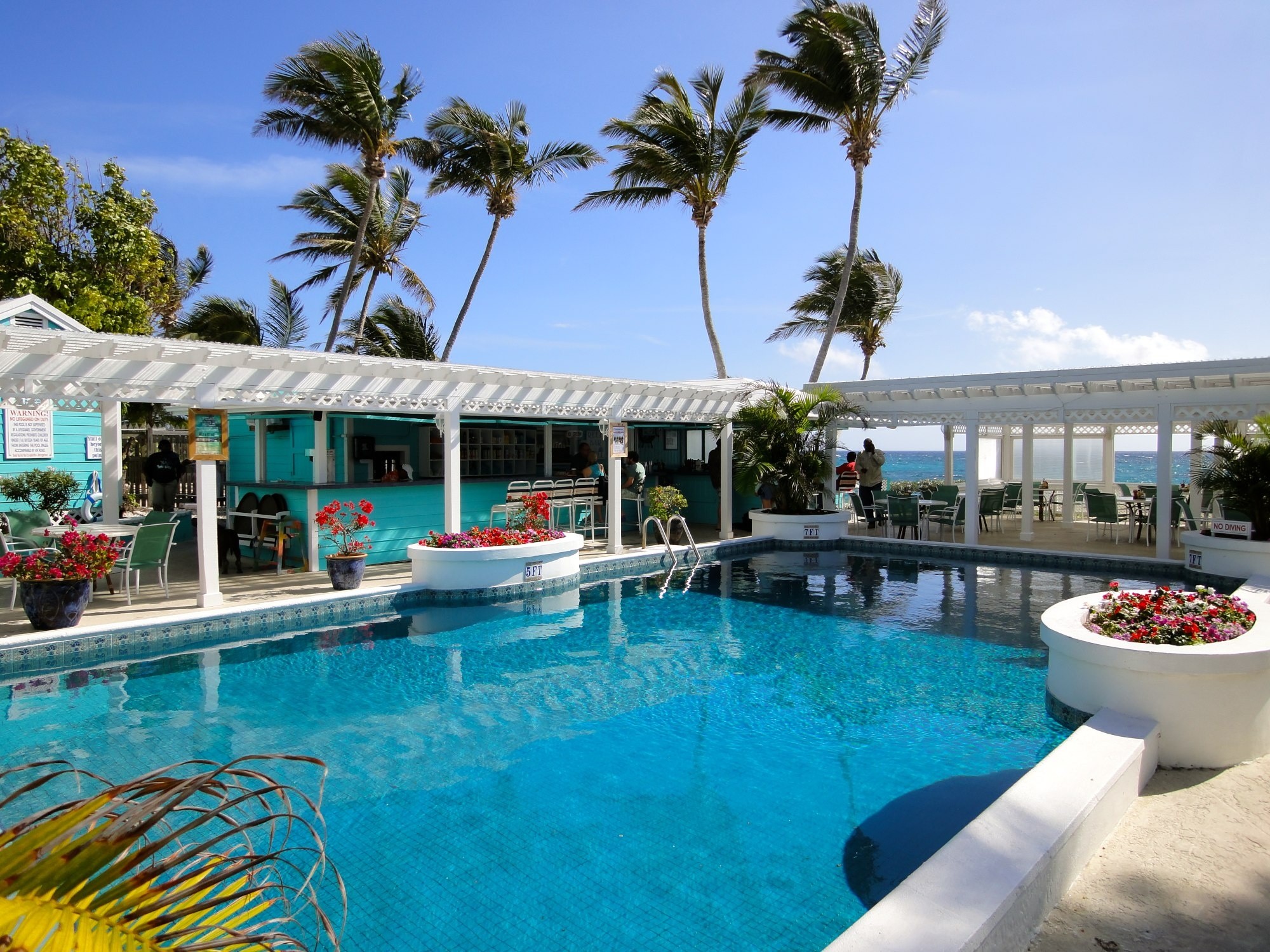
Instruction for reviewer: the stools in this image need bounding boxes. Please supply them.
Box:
[489,477,645,543]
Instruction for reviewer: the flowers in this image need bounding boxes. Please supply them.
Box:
[314,498,376,556]
[506,491,550,531]
[1,515,126,584]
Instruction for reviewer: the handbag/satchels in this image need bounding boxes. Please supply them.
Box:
[595,464,609,506]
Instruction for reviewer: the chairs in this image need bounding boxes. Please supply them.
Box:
[847,480,1214,542]
[0,509,181,605]
[835,471,858,510]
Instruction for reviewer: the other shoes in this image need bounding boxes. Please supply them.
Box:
[867,522,875,528]
[878,520,884,527]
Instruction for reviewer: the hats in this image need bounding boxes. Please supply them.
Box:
[402,464,414,480]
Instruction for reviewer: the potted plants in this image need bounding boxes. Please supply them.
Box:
[709,378,870,540]
[649,486,688,544]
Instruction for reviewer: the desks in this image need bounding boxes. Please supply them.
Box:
[1116,498,1152,544]
[1033,489,1065,520]
[31,524,137,594]
[875,499,948,542]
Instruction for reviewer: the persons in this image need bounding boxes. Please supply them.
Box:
[708,438,721,529]
[583,452,605,525]
[145,439,181,512]
[855,438,884,528]
[836,451,856,491]
[571,442,590,469]
[621,451,645,521]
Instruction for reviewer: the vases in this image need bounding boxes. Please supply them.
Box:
[324,553,368,591]
[16,577,92,629]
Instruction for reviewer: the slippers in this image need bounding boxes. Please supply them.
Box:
[595,520,603,524]
[583,516,592,523]
